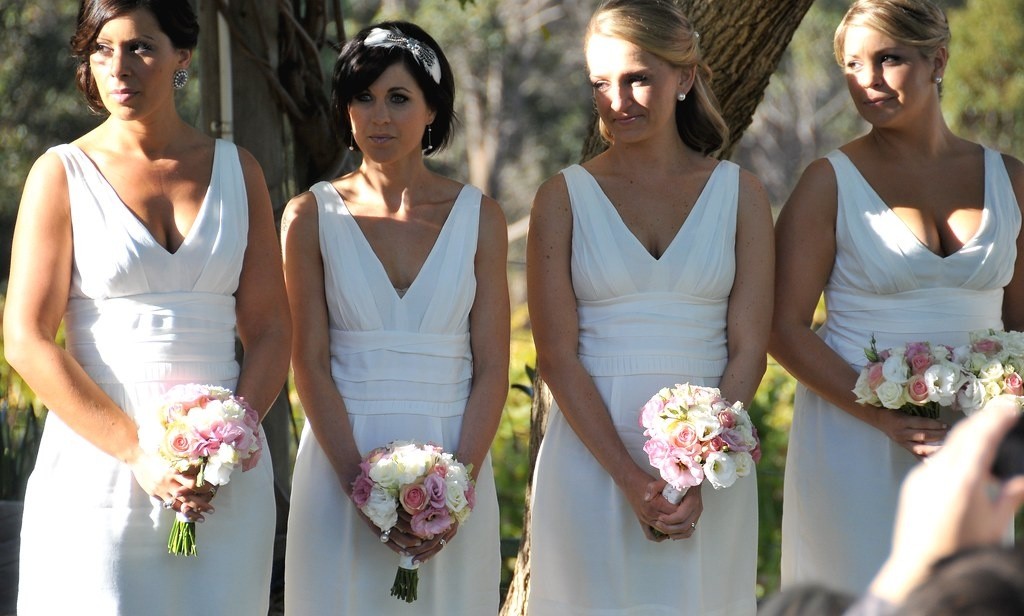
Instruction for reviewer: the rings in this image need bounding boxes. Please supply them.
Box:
[440,537,448,550]
[206,484,218,499]
[692,521,697,531]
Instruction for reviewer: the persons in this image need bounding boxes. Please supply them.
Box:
[503,0,777,615]
[1,1,292,615]
[278,21,514,616]
[758,0,1024,616]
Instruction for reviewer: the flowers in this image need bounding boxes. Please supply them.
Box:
[140,383,263,556]
[638,379,763,541]
[352,438,478,603]
[946,329,1023,421]
[851,328,968,457]
